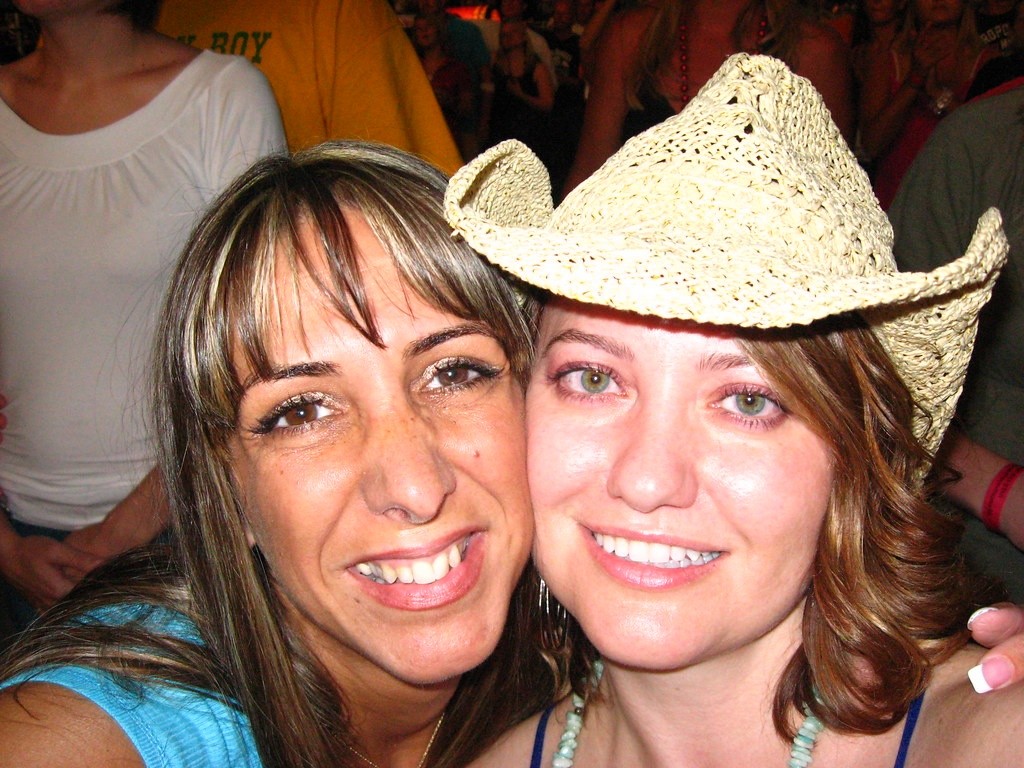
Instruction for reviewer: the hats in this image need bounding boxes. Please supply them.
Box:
[440,49,1010,503]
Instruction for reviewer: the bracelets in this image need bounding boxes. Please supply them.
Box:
[909,76,924,83]
[906,81,922,91]
[980,462,1024,530]
[930,89,953,114]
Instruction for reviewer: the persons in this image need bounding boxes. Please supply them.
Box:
[0,0,291,622]
[0,138,1024,768]
[889,88,1023,602]
[456,56,1024,767]
[153,1,463,179]
[388,0,1023,208]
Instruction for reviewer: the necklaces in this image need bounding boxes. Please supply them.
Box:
[678,19,768,100]
[554,660,824,768]
[342,712,445,767]
